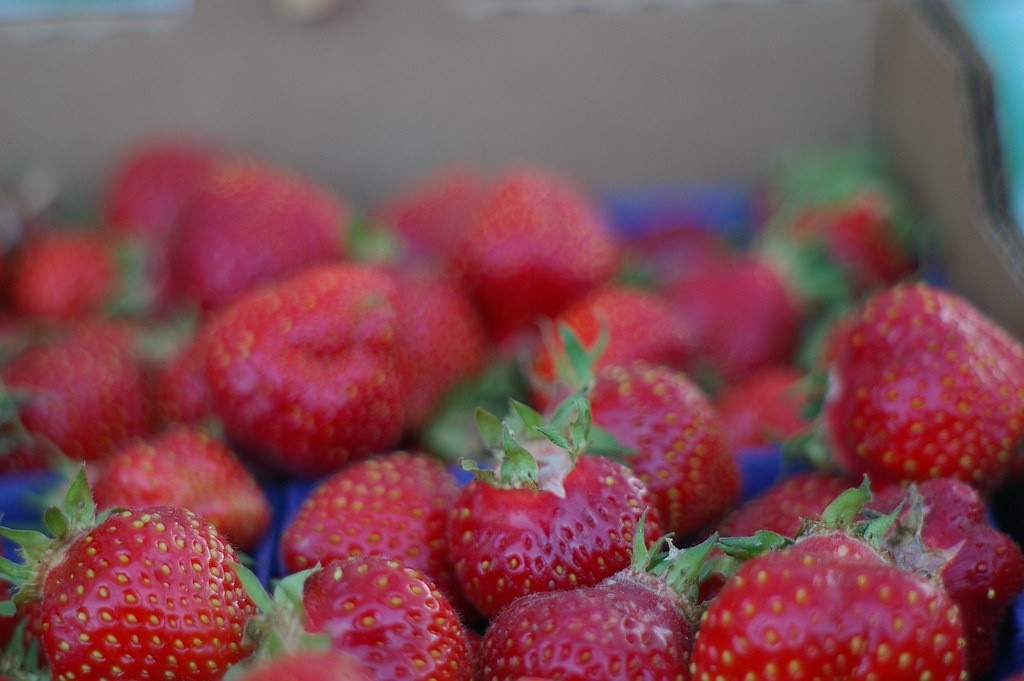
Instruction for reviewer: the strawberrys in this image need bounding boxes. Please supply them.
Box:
[1,136,1024,681]
